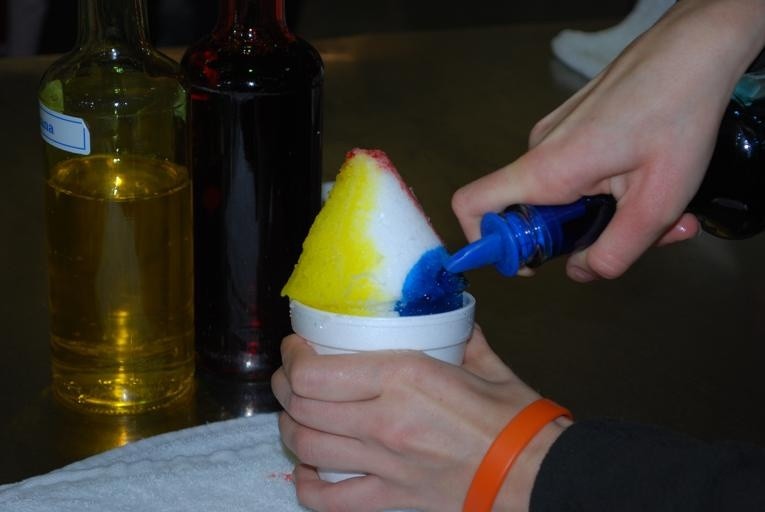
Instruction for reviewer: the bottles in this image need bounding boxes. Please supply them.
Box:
[35,0,202,417]
[443,68,765,277]
[171,0,328,391]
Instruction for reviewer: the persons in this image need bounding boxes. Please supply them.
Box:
[268,3,765,510]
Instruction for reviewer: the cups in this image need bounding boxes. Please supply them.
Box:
[288,288,477,485]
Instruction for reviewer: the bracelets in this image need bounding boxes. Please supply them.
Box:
[464,396,574,512]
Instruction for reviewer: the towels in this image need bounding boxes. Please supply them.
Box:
[0,411,306,511]
